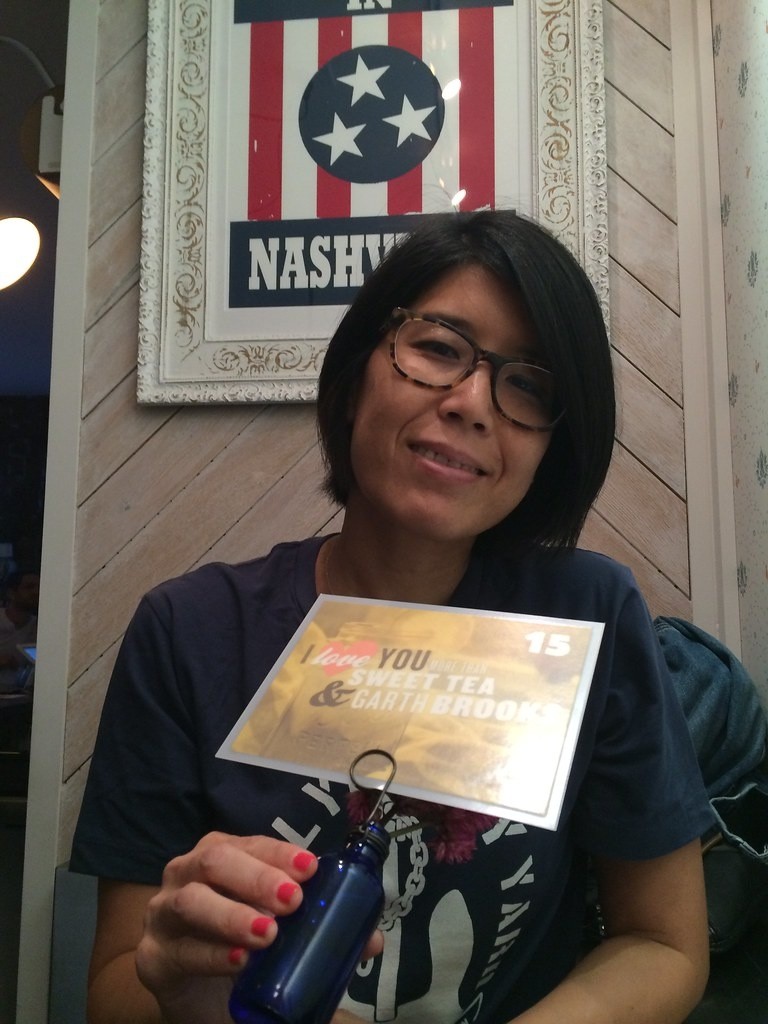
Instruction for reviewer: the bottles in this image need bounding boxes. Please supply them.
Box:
[227,820,391,1023]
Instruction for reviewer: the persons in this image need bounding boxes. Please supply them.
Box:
[0,568,40,692]
[64,209,712,1024]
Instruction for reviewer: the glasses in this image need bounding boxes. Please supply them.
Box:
[378,303,568,432]
